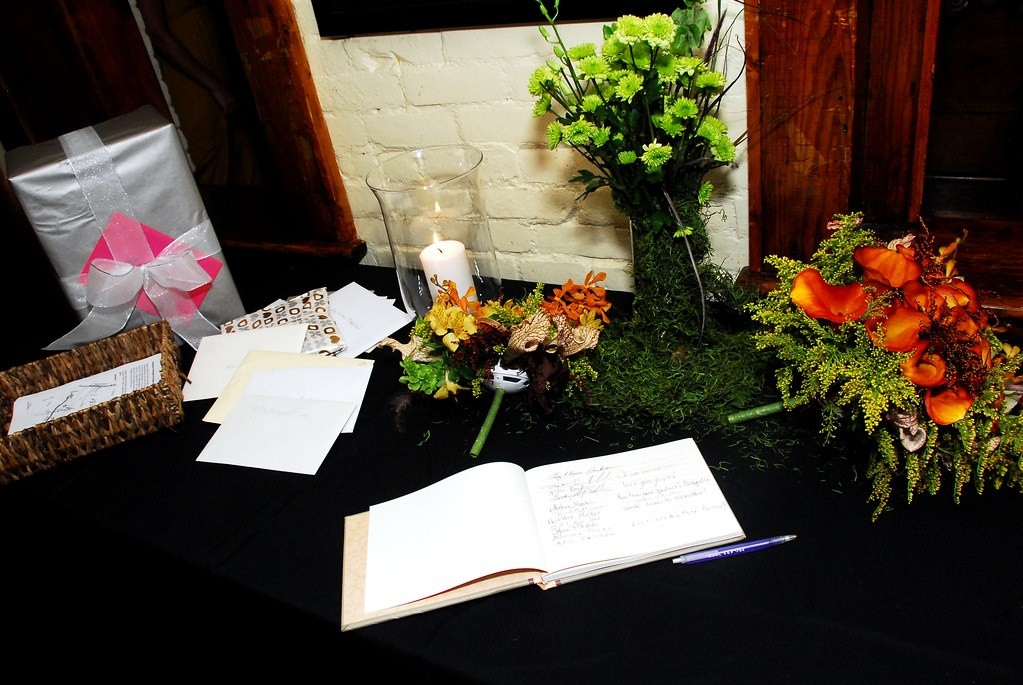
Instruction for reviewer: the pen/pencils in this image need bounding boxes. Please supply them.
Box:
[671,534,799,565]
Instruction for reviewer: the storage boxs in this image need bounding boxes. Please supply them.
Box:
[0,319,185,487]
[0,106,246,346]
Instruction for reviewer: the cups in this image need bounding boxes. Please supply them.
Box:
[368,144,503,323]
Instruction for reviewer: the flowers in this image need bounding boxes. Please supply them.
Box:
[525,0,742,241]
[728,205,1023,523]
[379,269,611,458]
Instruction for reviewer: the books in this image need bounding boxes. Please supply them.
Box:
[340,438,744,632]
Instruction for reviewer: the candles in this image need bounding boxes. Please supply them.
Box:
[419,232,478,302]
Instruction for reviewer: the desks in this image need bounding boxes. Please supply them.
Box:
[0,243,1023,685]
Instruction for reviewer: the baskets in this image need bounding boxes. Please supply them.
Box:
[0,319,184,486]
